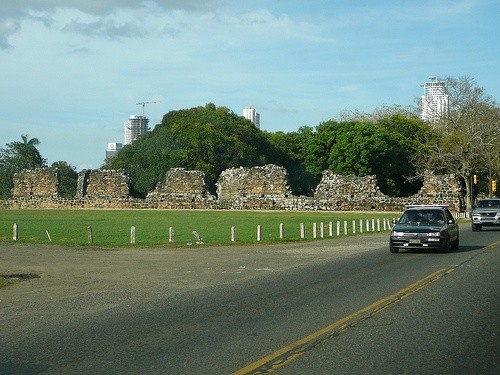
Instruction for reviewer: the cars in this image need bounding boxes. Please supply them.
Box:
[389,206,460,253]
[470,198,500,232]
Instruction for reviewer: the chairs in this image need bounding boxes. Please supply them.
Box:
[430,213,443,221]
